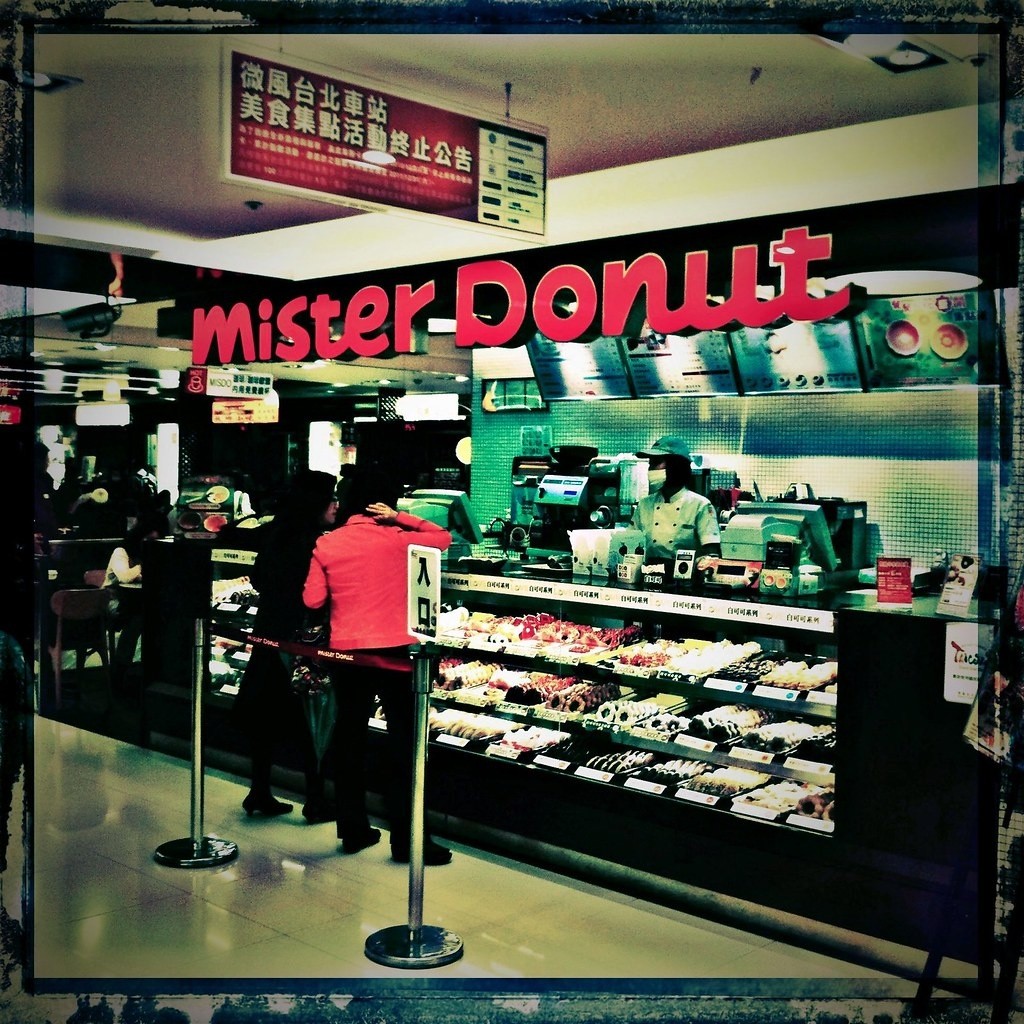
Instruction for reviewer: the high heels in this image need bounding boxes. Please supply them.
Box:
[243,798,293,816]
[302,802,338,824]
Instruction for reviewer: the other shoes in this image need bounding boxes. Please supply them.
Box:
[341,825,381,854]
[111,664,131,698]
[392,840,452,865]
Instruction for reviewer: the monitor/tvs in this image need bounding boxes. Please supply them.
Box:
[412,489,484,545]
[738,504,837,572]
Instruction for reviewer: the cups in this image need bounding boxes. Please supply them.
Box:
[826,270,983,387]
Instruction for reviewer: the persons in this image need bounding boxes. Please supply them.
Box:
[305,472,453,866]
[38,485,171,708]
[231,469,339,824]
[628,437,721,561]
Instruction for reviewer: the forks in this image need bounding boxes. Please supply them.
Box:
[185,491,214,504]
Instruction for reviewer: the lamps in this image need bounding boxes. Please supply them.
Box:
[59,295,124,340]
[103,349,121,402]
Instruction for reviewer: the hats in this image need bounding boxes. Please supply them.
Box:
[635,436,692,463]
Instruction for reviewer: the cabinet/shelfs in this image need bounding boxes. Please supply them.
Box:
[187,548,843,850]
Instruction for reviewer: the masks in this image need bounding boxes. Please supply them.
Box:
[648,469,666,492]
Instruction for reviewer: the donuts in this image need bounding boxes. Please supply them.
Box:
[430,612,835,823]
[930,323,968,359]
[886,320,921,356]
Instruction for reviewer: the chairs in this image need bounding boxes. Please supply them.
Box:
[45,587,110,710]
[84,567,122,681]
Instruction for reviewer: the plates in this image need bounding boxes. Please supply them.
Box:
[205,486,230,504]
[177,513,228,532]
